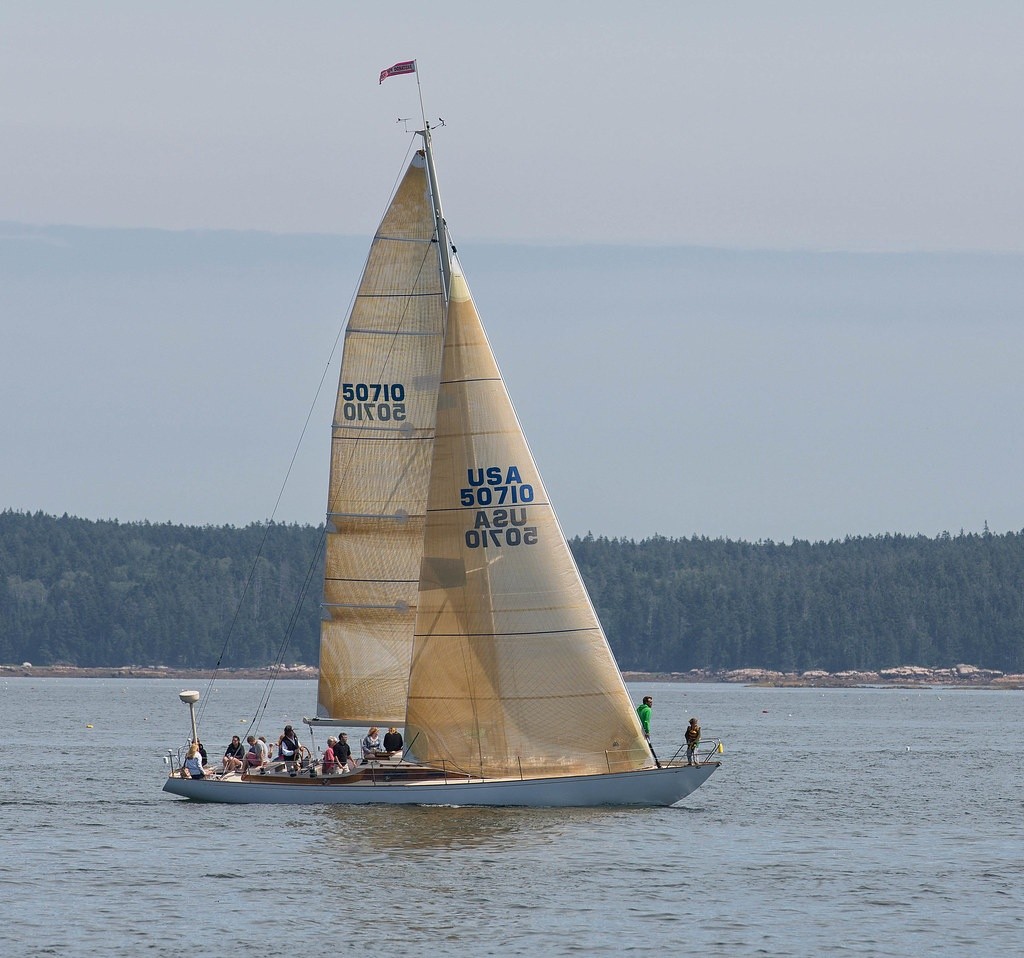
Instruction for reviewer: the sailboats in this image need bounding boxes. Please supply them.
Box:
[163,57,724,807]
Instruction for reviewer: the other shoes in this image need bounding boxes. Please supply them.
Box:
[695,761,700,765]
[687,762,692,765]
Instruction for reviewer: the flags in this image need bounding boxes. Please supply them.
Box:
[380,61,414,83]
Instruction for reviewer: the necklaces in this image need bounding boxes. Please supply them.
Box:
[282,727,304,761]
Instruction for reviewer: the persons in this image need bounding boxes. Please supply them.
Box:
[383,728,404,755]
[685,719,701,766]
[278,734,286,761]
[333,733,357,769]
[323,736,338,767]
[192,738,207,766]
[184,744,212,779]
[249,736,266,752]
[636,696,652,739]
[223,736,246,770]
[243,736,267,771]
[362,727,382,754]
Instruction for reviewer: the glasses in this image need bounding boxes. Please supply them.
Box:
[233,740,238,742]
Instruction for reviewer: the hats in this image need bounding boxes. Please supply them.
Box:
[689,718,697,724]
[284,725,292,733]
